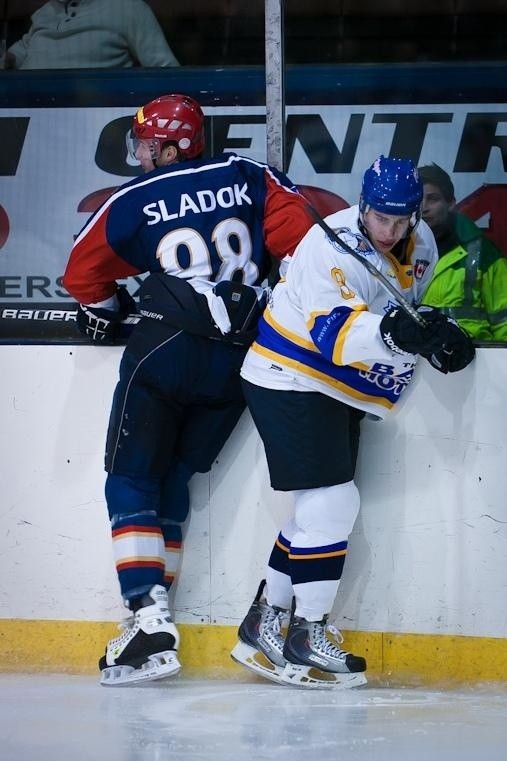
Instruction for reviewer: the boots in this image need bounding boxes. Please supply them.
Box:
[281,593,367,675]
[237,579,292,669]
[98,581,181,673]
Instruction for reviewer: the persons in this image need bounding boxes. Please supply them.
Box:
[0,1,187,69]
[60,91,320,670]
[419,161,506,344]
[234,156,478,675]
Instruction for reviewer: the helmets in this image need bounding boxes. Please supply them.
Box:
[358,154,423,241]
[130,94,208,161]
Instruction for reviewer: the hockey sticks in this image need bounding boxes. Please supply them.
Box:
[304,202,428,328]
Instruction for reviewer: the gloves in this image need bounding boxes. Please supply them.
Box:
[379,303,476,375]
[76,287,136,341]
